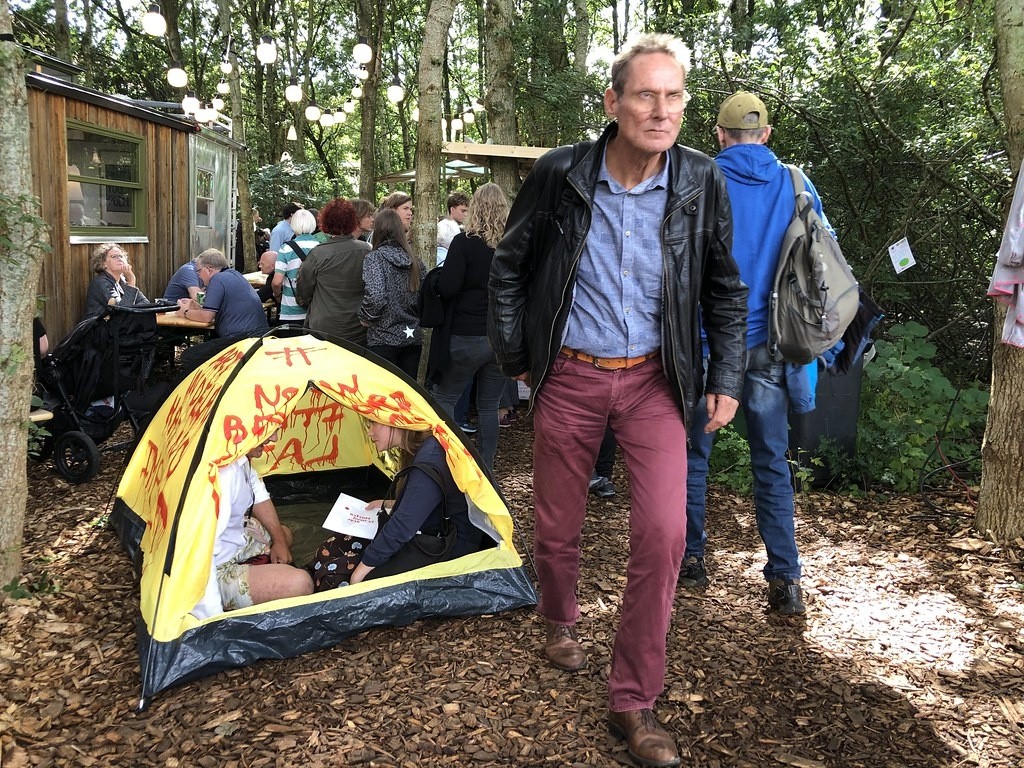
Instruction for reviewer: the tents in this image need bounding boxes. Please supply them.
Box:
[104,323,538,709]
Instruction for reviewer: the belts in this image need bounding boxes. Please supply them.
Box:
[560,346,659,373]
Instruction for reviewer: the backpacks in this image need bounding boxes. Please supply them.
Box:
[770,164,859,367]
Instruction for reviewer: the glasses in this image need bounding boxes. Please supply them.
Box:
[621,87,693,111]
[195,266,212,275]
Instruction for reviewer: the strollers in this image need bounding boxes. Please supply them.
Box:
[32,304,188,485]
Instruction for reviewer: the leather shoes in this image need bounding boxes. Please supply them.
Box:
[609,708,680,766]
[545,622,587,669]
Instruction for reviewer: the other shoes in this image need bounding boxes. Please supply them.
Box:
[456,421,477,432]
[499,409,516,428]
[588,471,616,498]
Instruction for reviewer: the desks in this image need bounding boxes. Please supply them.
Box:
[148,300,274,366]
[242,272,268,285]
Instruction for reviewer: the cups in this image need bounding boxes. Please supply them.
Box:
[197,292,206,306]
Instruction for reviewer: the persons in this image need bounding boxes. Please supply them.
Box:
[676,91,822,615]
[33,183,617,496]
[213,424,314,612]
[486,31,749,767]
[309,418,483,593]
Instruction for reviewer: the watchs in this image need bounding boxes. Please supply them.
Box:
[184,310,189,319]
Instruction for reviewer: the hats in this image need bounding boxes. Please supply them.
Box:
[714,90,768,130]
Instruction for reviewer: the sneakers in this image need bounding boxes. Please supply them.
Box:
[678,559,706,585]
[767,578,805,613]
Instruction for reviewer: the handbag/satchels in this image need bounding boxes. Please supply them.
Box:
[373,462,459,575]
